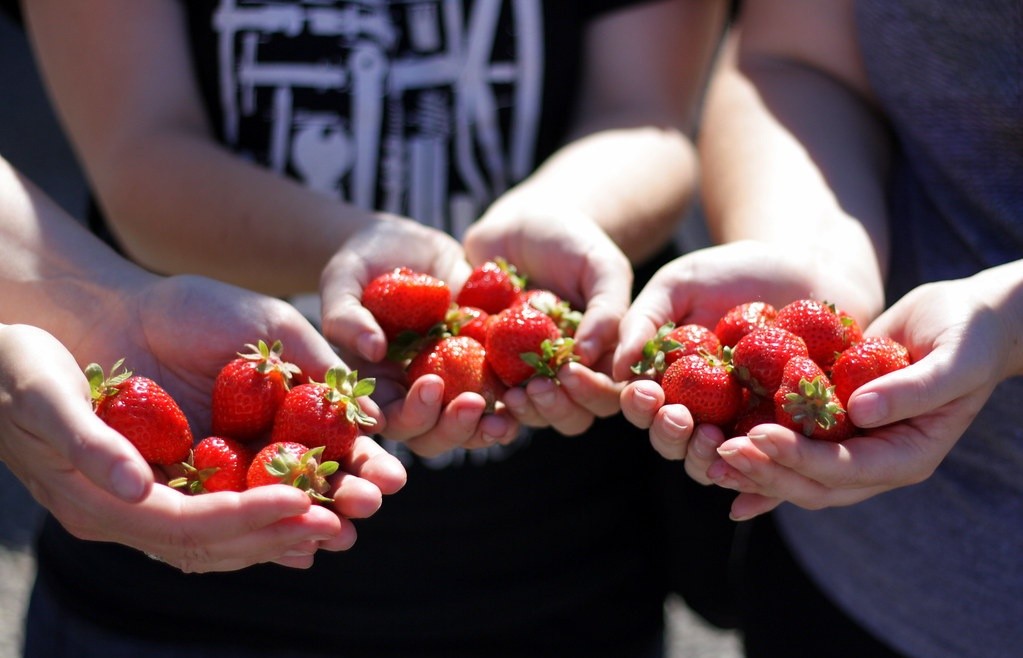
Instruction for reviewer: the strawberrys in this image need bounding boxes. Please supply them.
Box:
[84,260,910,508]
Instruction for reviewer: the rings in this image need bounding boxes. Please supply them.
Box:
[140,538,165,564]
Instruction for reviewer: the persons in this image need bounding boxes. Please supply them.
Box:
[9,0,737,658]
[0,147,409,578]
[613,0,1022,656]
